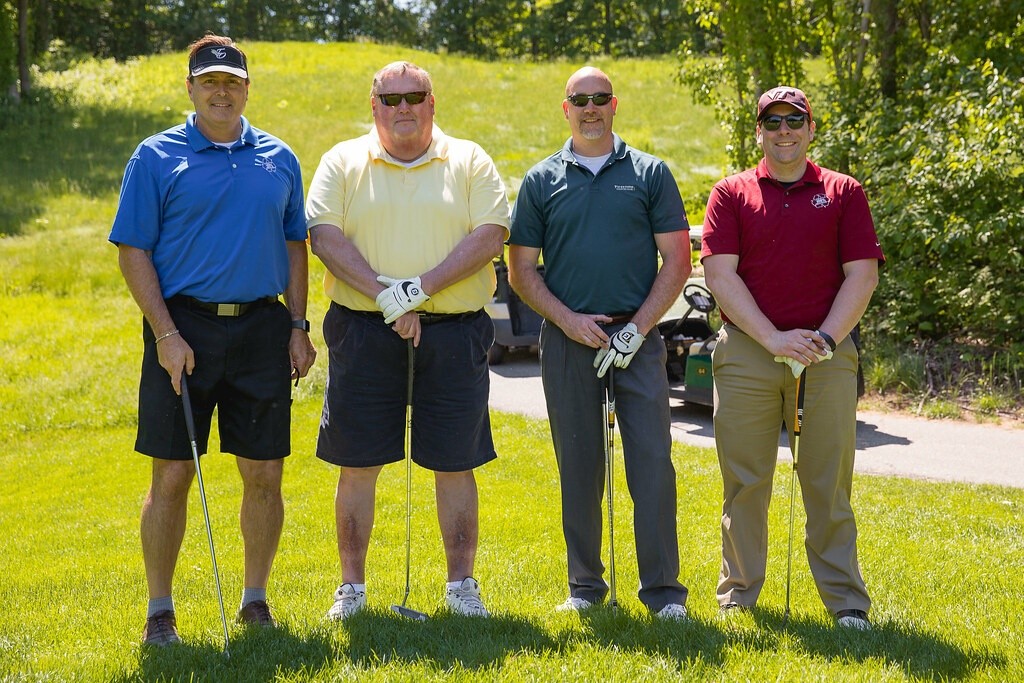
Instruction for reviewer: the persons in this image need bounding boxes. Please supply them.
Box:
[506,67,693,619]
[699,85,888,634]
[109,36,317,652]
[298,61,512,623]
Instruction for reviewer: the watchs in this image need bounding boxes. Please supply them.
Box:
[290,319,312,332]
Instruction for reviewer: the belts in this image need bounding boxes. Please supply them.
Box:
[596,312,635,326]
[175,293,267,316]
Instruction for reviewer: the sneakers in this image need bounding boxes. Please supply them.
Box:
[321,582,367,621]
[435,576,489,618]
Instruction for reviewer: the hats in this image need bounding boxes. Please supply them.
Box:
[188,45,248,79]
[756,86,812,124]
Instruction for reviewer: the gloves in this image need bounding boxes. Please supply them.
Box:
[593,322,646,378]
[375,275,431,324]
[773,329,833,379]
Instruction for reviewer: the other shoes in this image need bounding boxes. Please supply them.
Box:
[714,604,745,622]
[553,596,595,613]
[651,603,690,622]
[832,609,874,633]
[236,599,275,629]
[139,608,182,647]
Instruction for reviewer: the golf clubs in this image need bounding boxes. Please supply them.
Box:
[180,362,234,661]
[389,333,434,621]
[775,366,807,644]
[603,362,624,611]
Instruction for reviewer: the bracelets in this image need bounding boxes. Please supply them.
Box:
[813,327,837,355]
[155,329,179,344]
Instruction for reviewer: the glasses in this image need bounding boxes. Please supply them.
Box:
[374,91,430,106]
[567,93,613,106]
[759,112,809,131]
